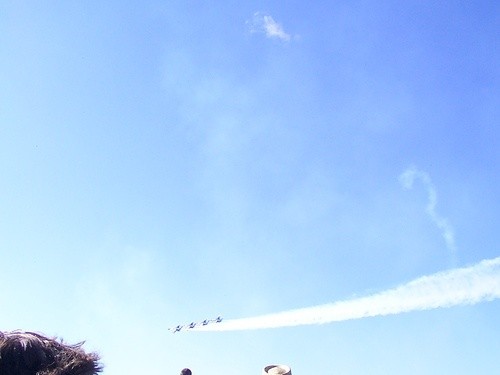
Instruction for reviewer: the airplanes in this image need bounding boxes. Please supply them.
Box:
[172,316,221,335]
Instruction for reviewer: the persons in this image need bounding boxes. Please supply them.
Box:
[262,364,293,375]
[179,368,192,375]
[1,329,105,375]
